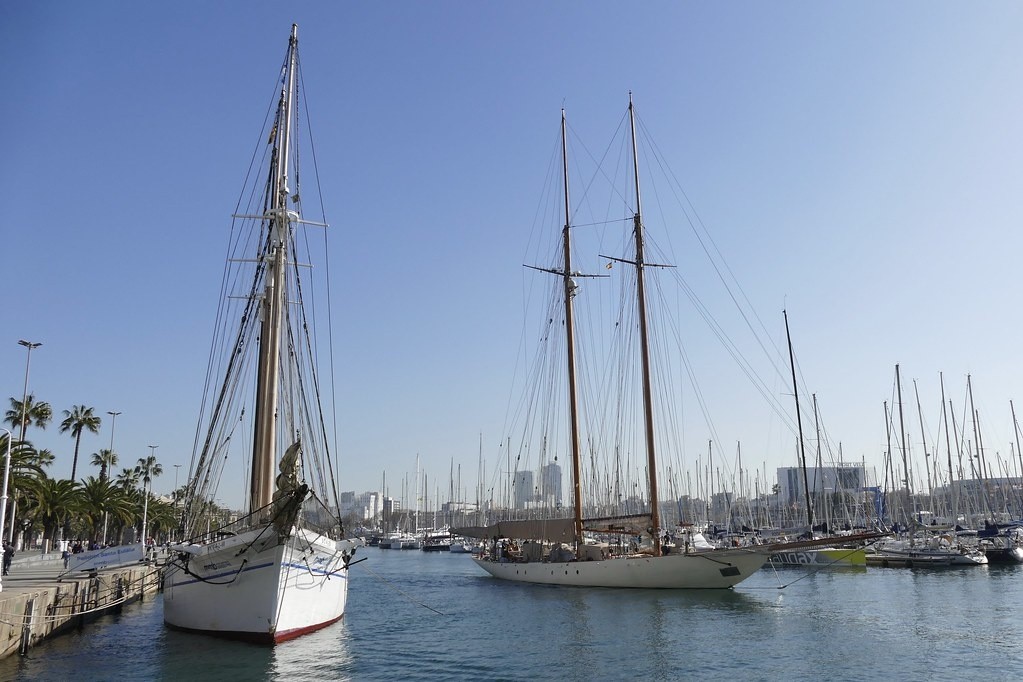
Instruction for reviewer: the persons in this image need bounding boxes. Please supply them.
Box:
[91,541,99,551]
[3,542,15,576]
[72,540,81,554]
[60,546,71,568]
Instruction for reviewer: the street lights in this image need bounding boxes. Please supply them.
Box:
[172,463,182,542]
[101,412,123,547]
[146,443,160,545]
[8,339,44,543]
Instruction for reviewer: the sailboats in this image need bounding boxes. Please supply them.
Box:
[470,89,896,590]
[162,22,365,650]
[370,311,1023,567]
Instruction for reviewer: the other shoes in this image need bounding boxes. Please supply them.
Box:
[3,571,8,576]
